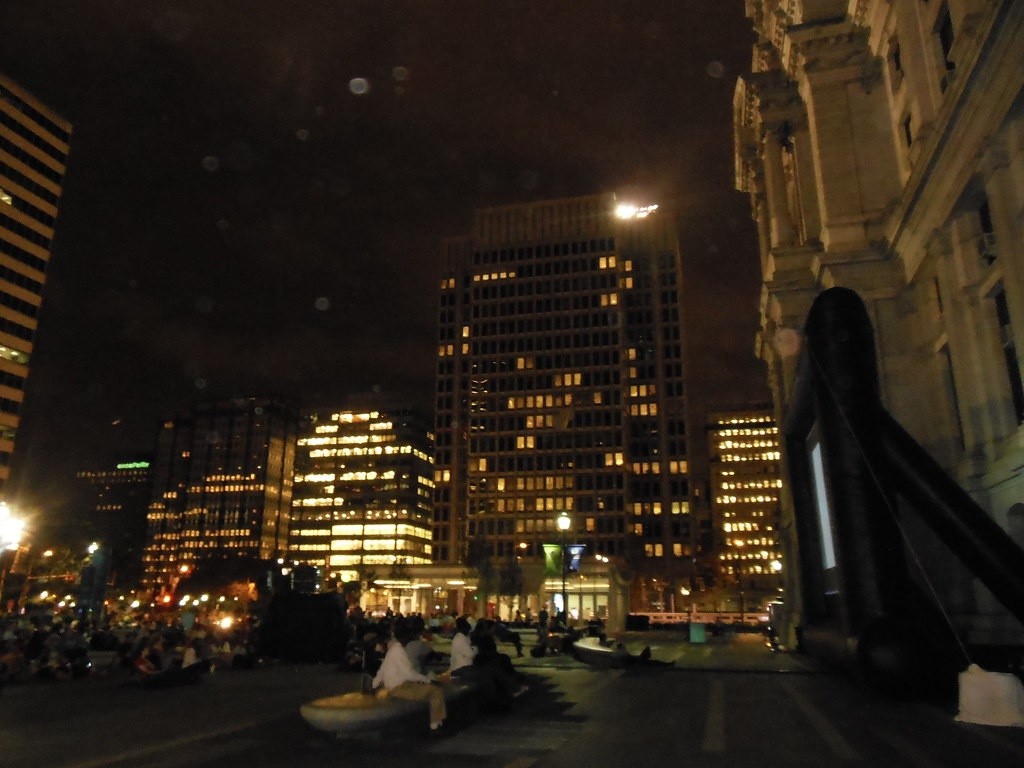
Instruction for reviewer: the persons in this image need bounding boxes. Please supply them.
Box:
[452,620,529,701]
[526,608,532,620]
[367,607,452,736]
[0,614,212,687]
[514,610,522,622]
[450,610,472,638]
[538,608,548,623]
[600,633,626,650]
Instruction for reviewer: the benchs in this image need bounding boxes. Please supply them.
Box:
[300,675,469,742]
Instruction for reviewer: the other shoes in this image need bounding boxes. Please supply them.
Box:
[512,685,529,698]
[427,724,445,737]
[518,653,525,657]
